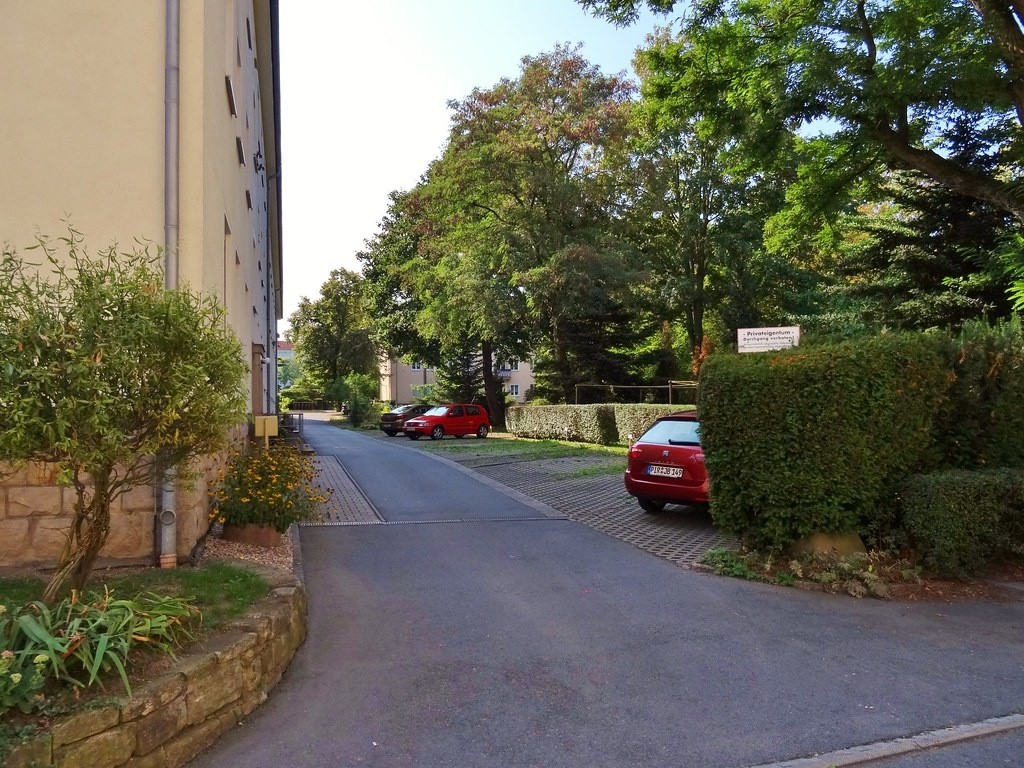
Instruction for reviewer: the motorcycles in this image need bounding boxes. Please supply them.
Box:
[341,401,349,415]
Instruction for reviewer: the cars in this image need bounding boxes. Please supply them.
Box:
[624,409,711,513]
[380,404,435,437]
[402,404,490,440]
